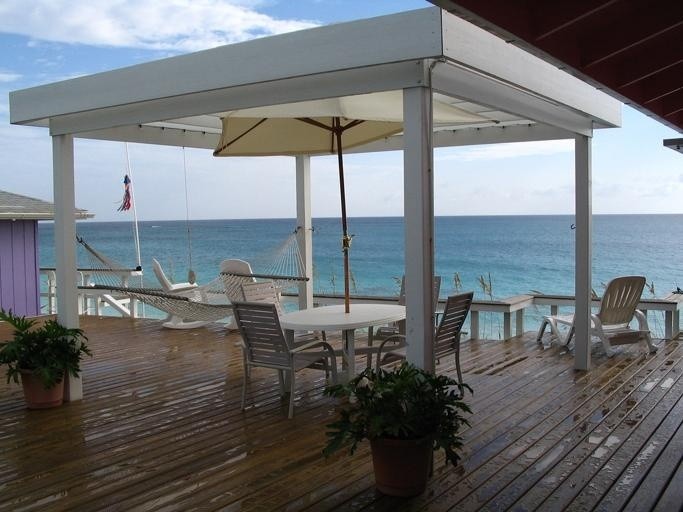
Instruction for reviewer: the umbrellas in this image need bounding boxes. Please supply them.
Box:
[213,88,502,312]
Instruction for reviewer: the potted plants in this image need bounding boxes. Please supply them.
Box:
[1,306,96,411]
[320,360,475,498]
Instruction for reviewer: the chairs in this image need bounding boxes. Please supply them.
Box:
[537,276,661,358]
[231,270,474,417]
